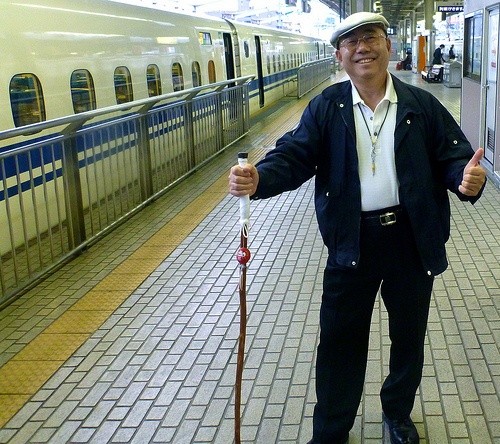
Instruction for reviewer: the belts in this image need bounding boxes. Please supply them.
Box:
[362,208,406,227]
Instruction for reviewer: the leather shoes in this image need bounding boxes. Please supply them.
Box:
[381,407,421,443]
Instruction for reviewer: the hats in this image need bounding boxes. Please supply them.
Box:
[329,12,390,50]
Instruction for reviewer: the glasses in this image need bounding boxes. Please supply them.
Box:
[339,32,389,50]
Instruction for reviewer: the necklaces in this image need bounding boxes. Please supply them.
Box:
[357,101,396,175]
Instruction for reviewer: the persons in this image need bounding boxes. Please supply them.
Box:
[230,11,487,444]
[449,44,455,59]
[402,51,412,70]
[433,44,445,65]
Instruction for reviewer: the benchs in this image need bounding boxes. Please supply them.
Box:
[422,66,444,83]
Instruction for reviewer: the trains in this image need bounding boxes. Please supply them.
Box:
[0,0,336,261]
